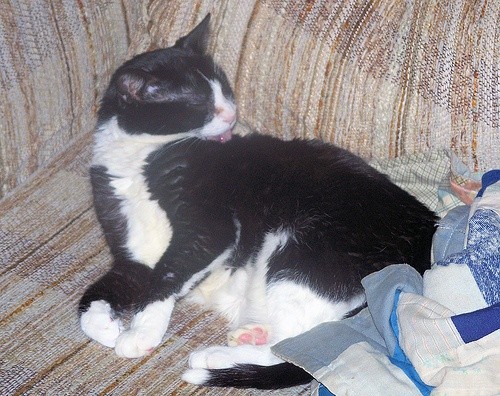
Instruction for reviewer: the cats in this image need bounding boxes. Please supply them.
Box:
[77,11,442,390]
